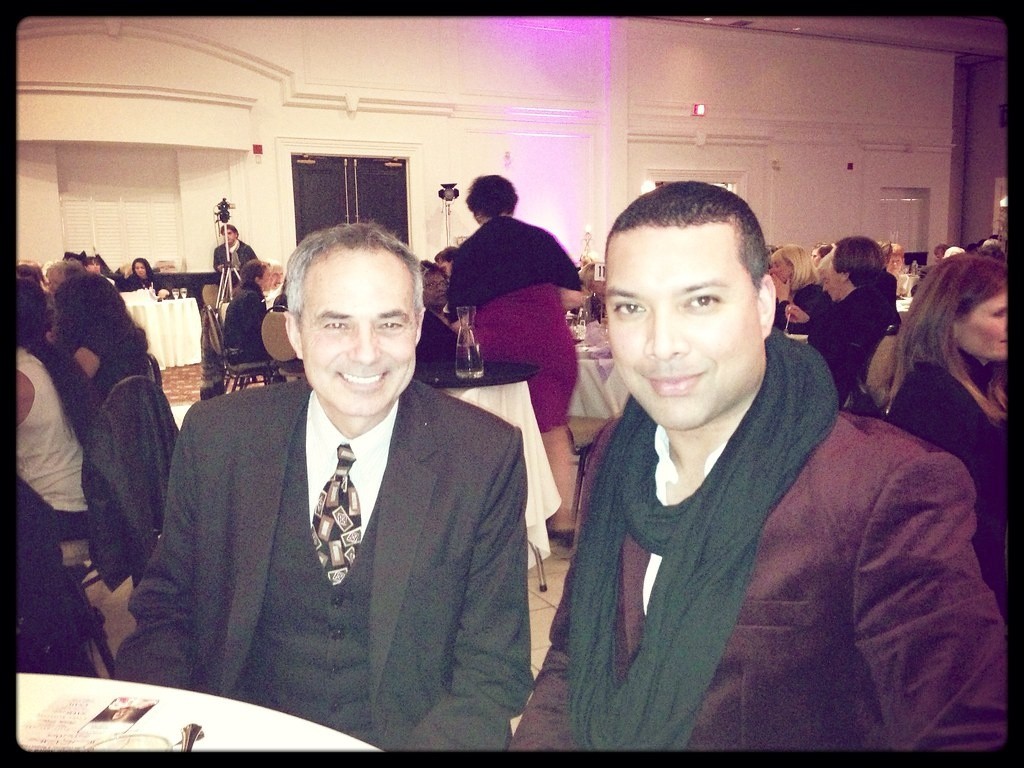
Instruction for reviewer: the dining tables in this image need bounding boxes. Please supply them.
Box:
[121,288,202,371]
[566,341,629,455]
[442,382,562,593]
[16,672,391,752]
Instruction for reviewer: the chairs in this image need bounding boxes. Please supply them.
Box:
[207,305,271,394]
[260,305,305,382]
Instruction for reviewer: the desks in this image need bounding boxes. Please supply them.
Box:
[105,272,239,310]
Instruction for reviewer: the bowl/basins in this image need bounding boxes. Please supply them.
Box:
[576,346,597,358]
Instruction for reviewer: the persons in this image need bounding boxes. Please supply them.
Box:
[16,252,170,674]
[213,224,287,383]
[768,234,1009,623]
[416,175,605,549]
[116,222,535,753]
[504,181,1009,753]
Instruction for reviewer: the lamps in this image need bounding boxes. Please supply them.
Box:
[344,89,360,113]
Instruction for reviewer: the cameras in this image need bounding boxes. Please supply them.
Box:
[217,211,232,223]
[216,197,236,210]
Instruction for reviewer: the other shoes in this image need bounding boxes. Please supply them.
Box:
[547,519,575,558]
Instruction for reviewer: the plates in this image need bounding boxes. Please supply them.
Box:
[88,733,173,751]
[788,334,810,339]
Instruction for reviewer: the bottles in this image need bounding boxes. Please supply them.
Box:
[454,305,485,379]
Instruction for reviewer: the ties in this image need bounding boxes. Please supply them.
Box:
[310,444,361,585]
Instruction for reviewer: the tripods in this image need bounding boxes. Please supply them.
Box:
[213,224,241,320]
